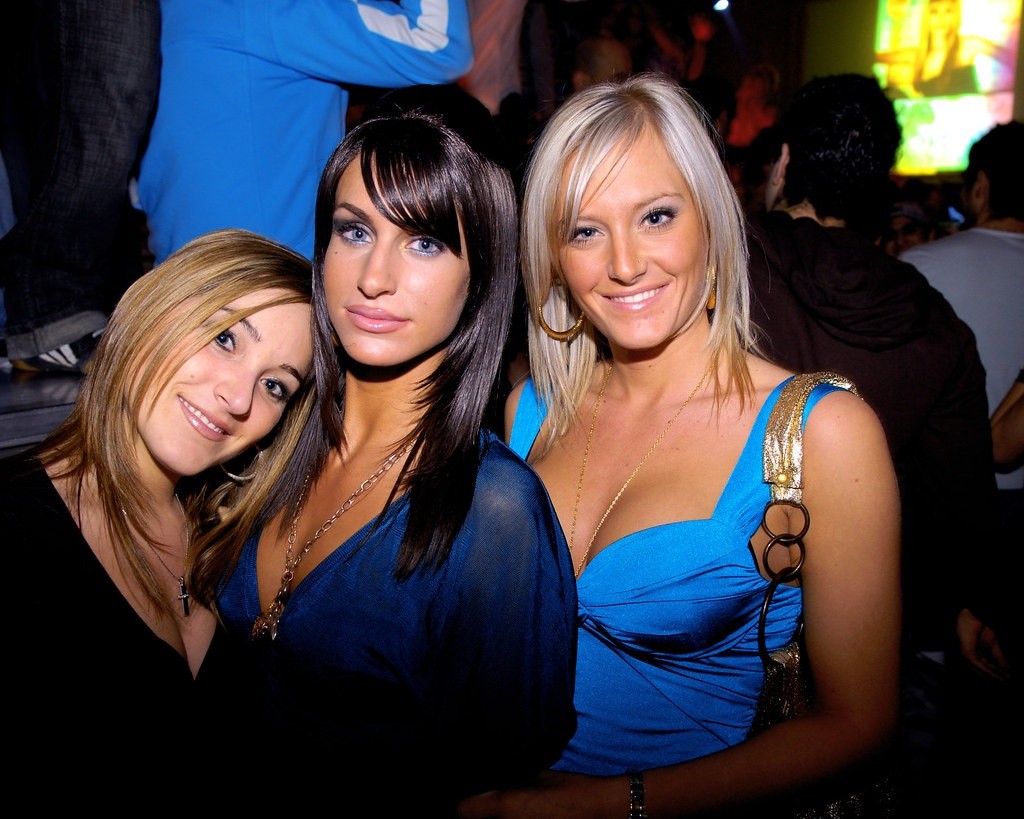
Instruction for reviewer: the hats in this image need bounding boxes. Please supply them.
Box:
[887,203,928,224]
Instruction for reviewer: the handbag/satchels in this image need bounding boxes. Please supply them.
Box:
[745,372,897,819]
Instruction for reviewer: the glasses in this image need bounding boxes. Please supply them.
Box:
[881,219,920,244]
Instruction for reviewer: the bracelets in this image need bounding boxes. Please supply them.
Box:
[628,772,648,819]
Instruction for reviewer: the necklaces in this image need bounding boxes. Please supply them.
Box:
[123,493,189,616]
[251,438,418,640]
[569,362,712,578]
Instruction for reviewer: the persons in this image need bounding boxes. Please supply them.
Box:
[136,0,475,265]
[0,228,315,819]
[990,369,1024,463]
[742,74,1024,685]
[897,121,1024,490]
[187,112,580,819]
[455,75,902,819]
[572,35,632,94]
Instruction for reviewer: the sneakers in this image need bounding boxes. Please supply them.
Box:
[8,327,107,375]
